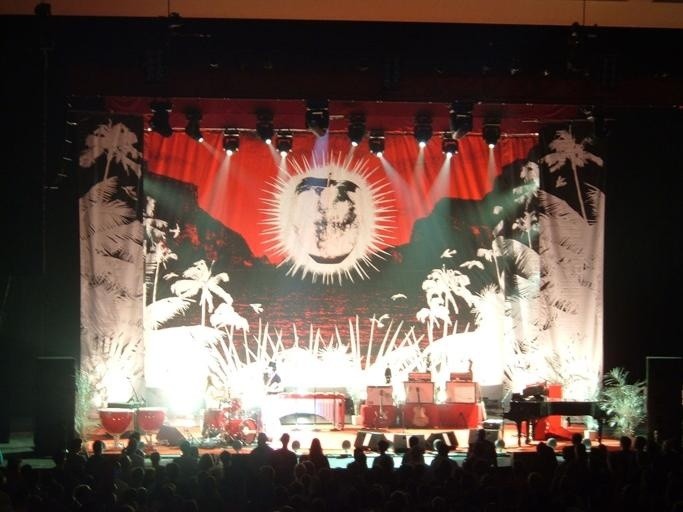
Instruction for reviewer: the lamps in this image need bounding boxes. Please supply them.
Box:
[222,129,239,157]
[481,118,500,150]
[368,129,385,156]
[184,110,204,143]
[304,104,329,137]
[413,114,432,149]
[347,116,365,147]
[255,109,274,144]
[450,103,473,137]
[149,102,175,137]
[442,132,458,157]
[276,130,293,156]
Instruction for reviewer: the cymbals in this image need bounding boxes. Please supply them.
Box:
[215,387,234,392]
[213,395,225,401]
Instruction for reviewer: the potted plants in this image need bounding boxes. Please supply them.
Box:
[593,365,649,447]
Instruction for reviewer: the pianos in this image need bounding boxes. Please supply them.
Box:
[500,387,607,447]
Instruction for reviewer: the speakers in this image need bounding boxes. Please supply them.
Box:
[646,357,683,441]
[33,356,76,454]
[468,428,499,448]
[354,431,459,453]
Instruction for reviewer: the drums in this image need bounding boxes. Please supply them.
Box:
[224,417,257,446]
[136,407,169,434]
[202,408,227,432]
[98,408,133,439]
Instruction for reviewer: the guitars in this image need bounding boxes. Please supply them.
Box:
[371,389,391,429]
[411,387,429,428]
